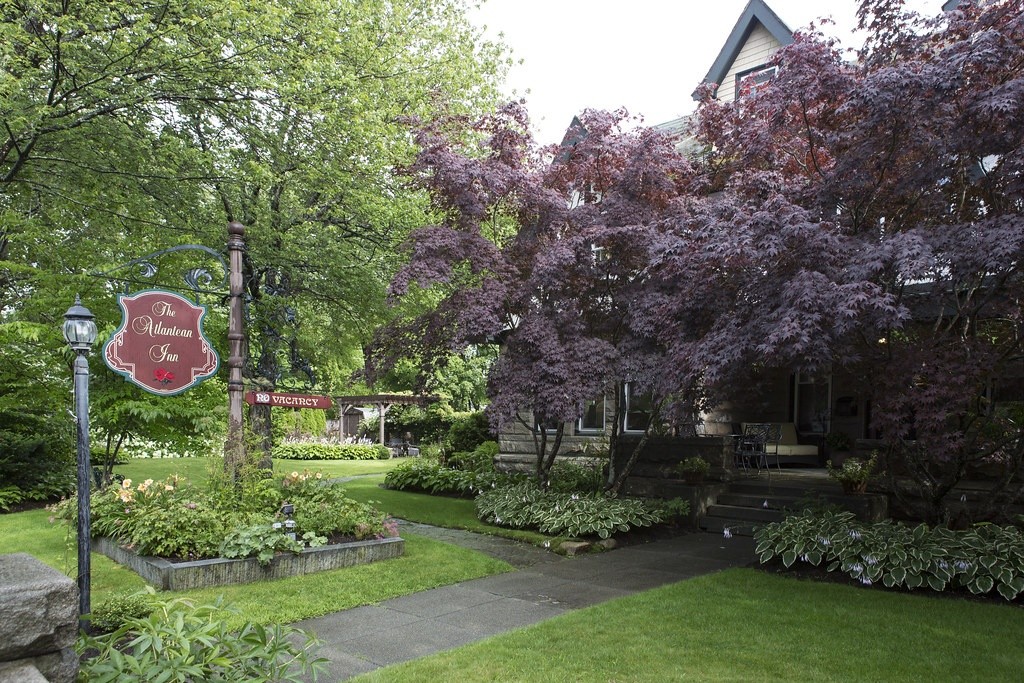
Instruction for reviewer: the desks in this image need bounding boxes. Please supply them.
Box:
[698,434,742,473]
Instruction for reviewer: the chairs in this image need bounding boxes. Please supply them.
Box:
[692,415,783,480]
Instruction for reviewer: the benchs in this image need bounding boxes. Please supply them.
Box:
[732,420,824,469]
